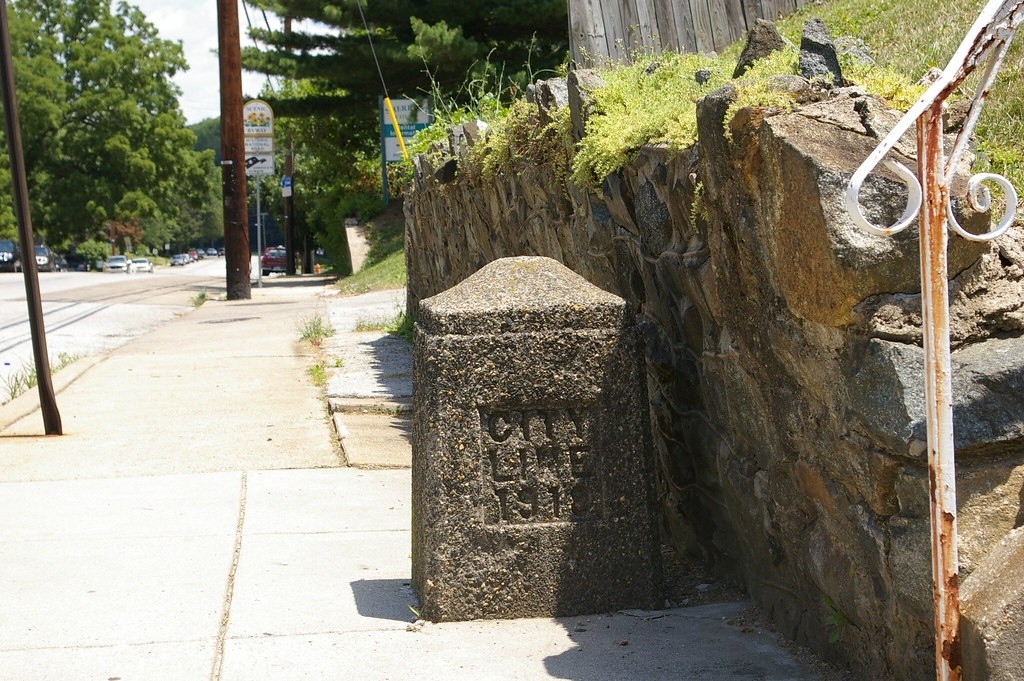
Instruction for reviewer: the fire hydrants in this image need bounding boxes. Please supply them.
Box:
[315,262,320,274]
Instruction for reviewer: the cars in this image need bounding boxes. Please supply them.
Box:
[129,257,154,273]
[218,247,225,257]
[53,253,64,271]
[207,248,217,256]
[261,246,287,275]
[65,254,89,271]
[171,248,206,267]
[102,256,132,273]
[33,246,56,272]
[0,240,23,273]
